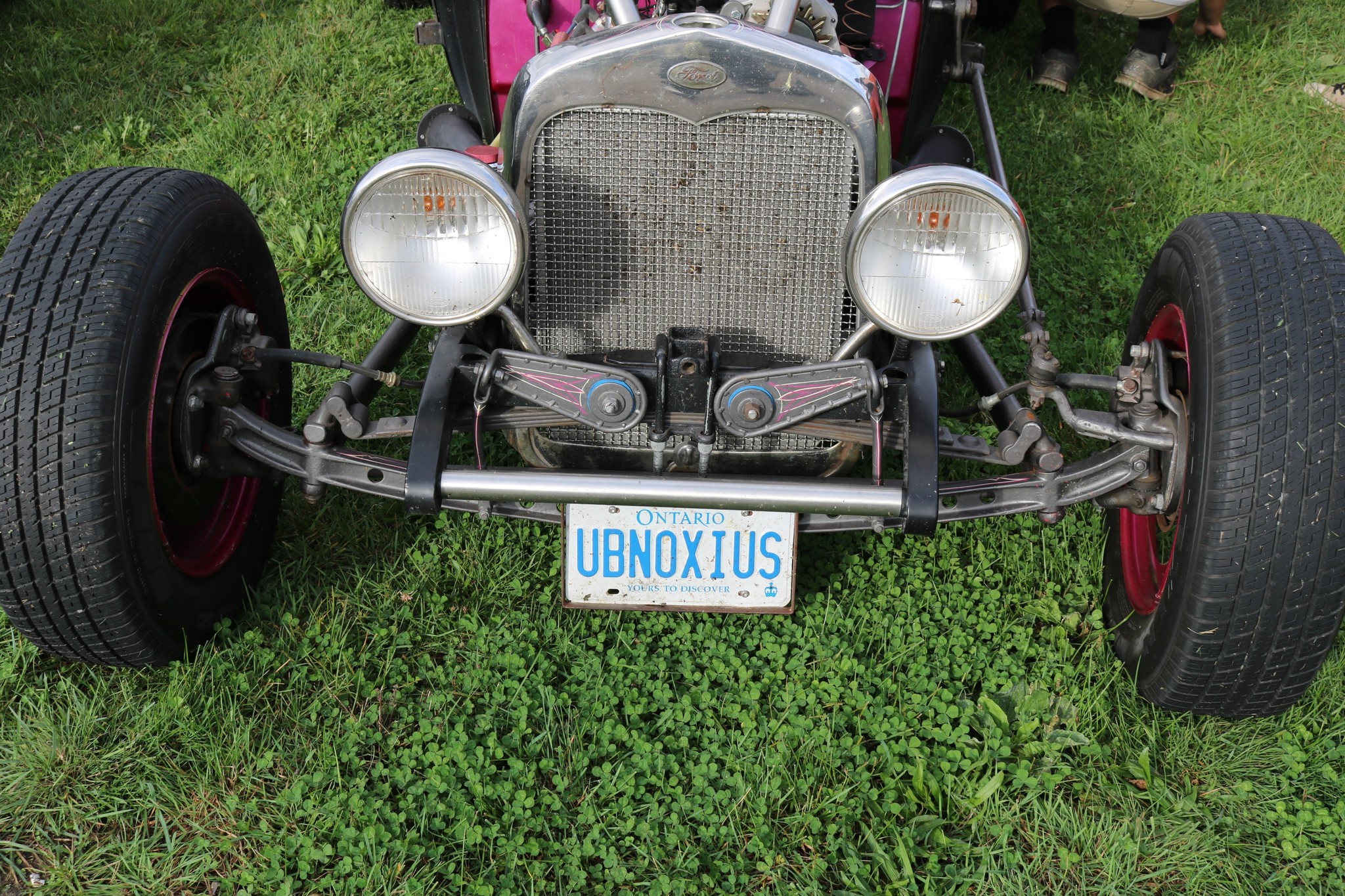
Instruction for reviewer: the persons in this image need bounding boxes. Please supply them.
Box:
[1029,0,1228,101]
[1305,80,1345,116]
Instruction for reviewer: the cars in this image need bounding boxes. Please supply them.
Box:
[1,1,1342,723]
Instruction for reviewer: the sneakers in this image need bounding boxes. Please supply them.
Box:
[1115,48,1176,103]
[1033,46,1080,93]
